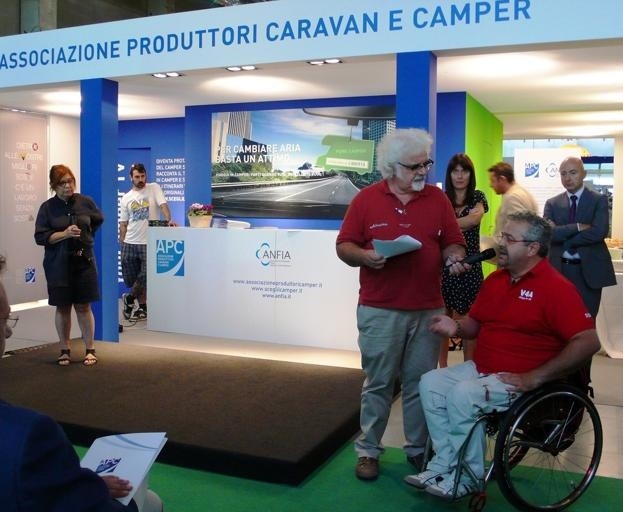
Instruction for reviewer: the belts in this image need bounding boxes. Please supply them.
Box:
[561,258,580,265]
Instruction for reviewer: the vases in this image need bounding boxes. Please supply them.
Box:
[188,216,212,228]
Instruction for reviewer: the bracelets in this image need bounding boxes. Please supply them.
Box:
[455,319,462,338]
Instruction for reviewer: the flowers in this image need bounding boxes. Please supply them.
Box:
[188,202,215,216]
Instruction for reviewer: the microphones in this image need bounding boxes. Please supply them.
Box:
[443,247,497,277]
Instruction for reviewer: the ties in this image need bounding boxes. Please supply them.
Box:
[568,196,577,254]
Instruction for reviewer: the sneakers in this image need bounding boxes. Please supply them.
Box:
[356,457,379,478]
[425,470,484,499]
[403,471,451,489]
[122,293,135,319]
[129,309,147,321]
[408,449,434,470]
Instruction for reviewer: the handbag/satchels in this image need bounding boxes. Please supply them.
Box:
[149,220,168,226]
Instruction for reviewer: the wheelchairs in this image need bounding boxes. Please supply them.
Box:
[417,305,603,511]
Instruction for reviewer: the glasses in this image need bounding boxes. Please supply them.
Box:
[396,158,433,171]
[0,316,18,329]
[57,178,73,186]
[131,164,144,168]
[496,235,541,245]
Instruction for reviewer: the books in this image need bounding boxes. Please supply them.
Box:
[82,431,168,507]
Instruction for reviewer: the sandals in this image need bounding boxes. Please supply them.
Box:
[84,349,97,366]
[57,349,71,366]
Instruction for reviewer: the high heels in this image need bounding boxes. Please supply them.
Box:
[447,338,463,350]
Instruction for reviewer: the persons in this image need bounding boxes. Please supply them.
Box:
[119,163,180,319]
[33,165,104,367]
[336,127,471,480]
[488,161,538,270]
[439,153,489,369]
[405,210,601,499]
[2,285,163,511]
[544,158,616,321]
